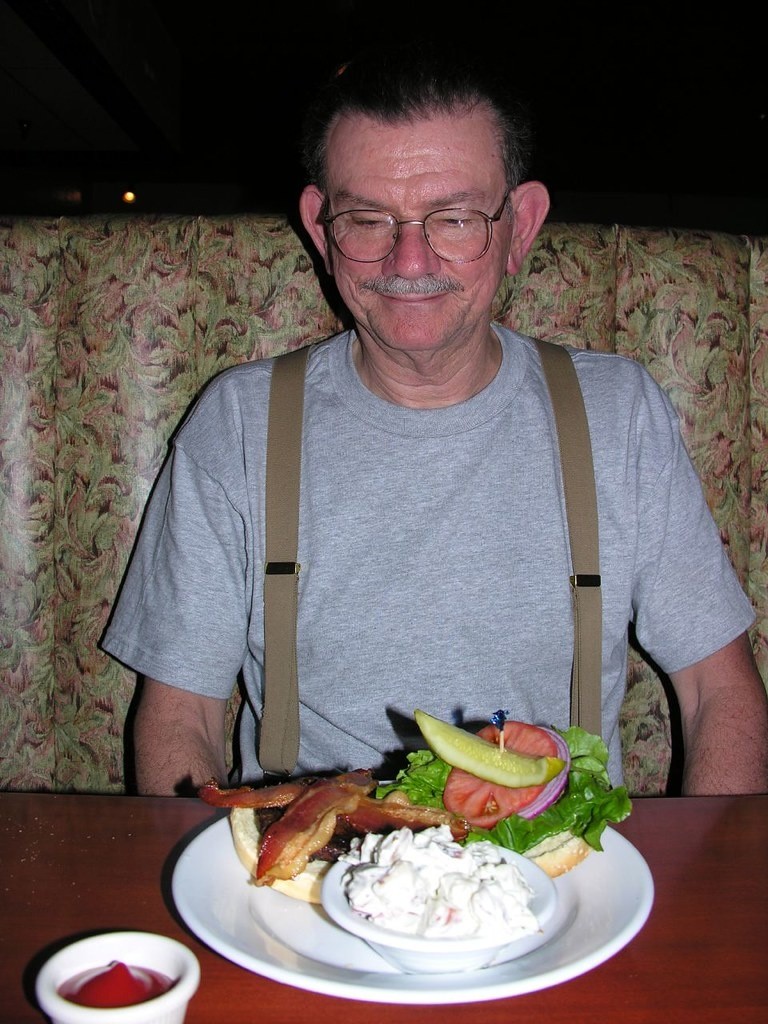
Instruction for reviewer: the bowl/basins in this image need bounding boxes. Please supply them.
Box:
[35,931,201,1024]
[319,841,559,976]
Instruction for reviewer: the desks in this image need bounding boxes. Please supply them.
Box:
[1,797,768,1024]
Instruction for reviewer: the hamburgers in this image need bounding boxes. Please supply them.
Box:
[198,706,634,906]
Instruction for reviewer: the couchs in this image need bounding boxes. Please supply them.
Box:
[4,215,768,796]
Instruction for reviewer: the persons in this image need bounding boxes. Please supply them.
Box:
[101,41,767,799]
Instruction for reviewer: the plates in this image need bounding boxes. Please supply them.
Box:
[171,779,655,1005]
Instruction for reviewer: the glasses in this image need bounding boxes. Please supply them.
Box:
[322,189,510,264]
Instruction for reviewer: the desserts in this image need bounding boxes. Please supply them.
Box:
[321,823,558,977]
